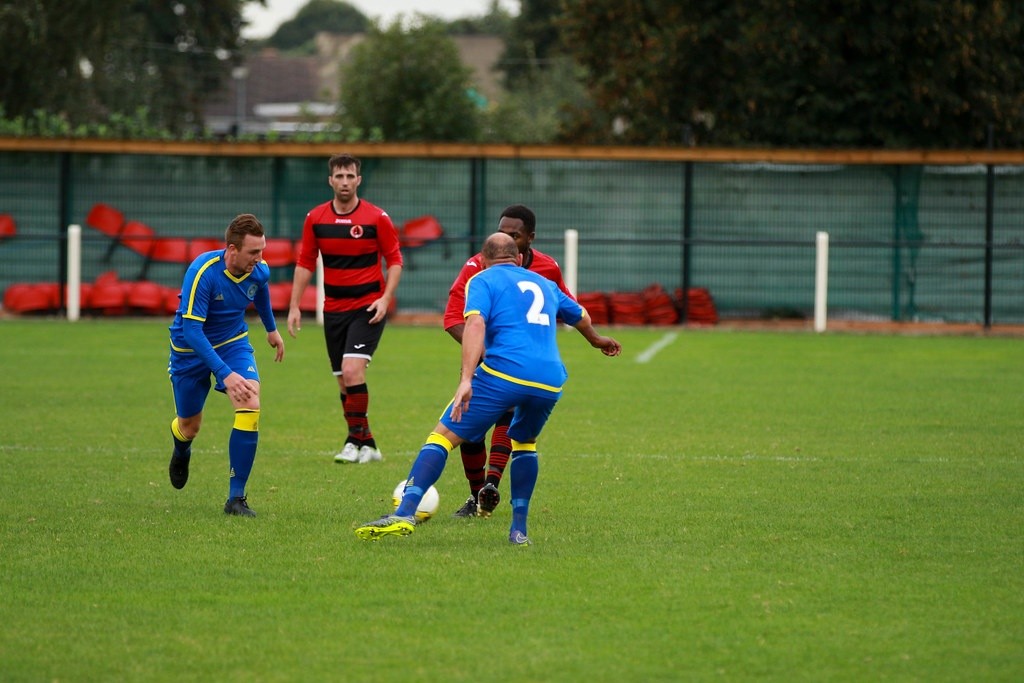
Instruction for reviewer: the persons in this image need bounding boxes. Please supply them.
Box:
[443,204,578,517]
[288,154,403,464]
[355,233,621,543]
[167,214,284,517]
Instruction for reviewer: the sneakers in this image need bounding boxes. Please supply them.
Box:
[223,493,256,517]
[359,445,382,464]
[509,530,530,546]
[355,515,416,542]
[169,447,190,489]
[452,494,478,519]
[334,442,358,464]
[477,483,501,519]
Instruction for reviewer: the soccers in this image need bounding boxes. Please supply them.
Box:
[392,477,439,525]
